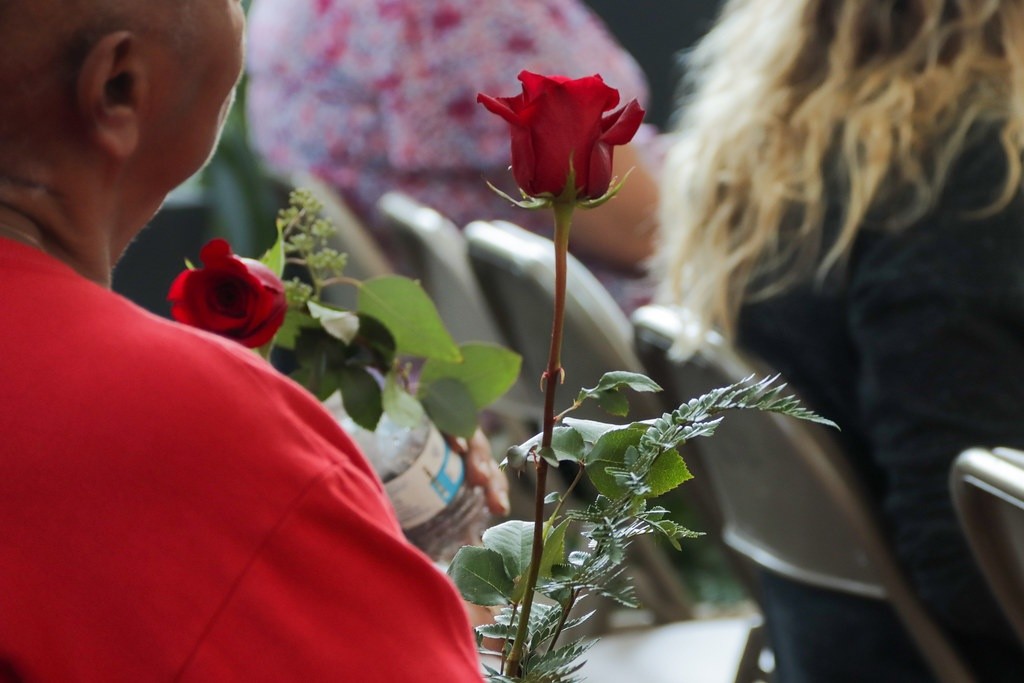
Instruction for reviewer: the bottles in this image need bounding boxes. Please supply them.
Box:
[319,368,492,573]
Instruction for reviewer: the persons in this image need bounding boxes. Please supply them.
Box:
[656,0,1023,683]
[0,1,487,682]
[244,0,664,316]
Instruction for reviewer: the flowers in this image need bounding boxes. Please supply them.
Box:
[444,69,843,682]
[167,185,525,440]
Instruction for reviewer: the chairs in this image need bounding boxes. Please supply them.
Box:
[462,217,665,634]
[949,445,1024,648]
[288,170,391,308]
[367,188,586,550]
[627,303,969,683]
